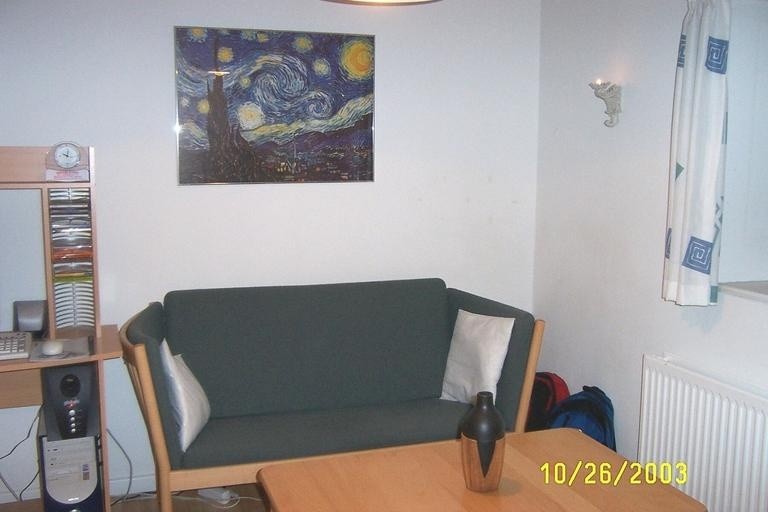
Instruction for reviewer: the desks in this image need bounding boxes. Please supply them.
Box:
[256,427,711,512]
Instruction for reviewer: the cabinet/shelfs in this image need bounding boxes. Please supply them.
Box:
[0,144,123,511]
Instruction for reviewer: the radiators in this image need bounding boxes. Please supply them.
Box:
[637,354,767,512]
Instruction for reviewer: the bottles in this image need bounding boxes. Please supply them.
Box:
[458,391,503,494]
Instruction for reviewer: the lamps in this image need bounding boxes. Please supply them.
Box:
[589,81,621,127]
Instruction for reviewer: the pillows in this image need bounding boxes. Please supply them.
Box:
[439,309,516,407]
[160,337,211,453]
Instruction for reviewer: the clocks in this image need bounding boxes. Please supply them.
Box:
[45,141,88,172]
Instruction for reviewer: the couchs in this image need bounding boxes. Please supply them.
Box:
[118,278,545,512]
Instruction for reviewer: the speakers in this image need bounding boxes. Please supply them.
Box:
[13,300,47,339]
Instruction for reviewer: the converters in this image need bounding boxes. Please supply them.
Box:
[197,487,231,502]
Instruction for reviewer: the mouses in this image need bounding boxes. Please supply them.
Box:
[42,340,64,357]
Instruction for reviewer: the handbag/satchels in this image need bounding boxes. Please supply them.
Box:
[542,385,617,452]
[526,371,570,430]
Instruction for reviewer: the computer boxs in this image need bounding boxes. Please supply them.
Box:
[34,394,109,512]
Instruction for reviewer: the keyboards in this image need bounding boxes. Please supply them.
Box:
[1,329,32,361]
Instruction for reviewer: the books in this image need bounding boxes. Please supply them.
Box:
[49,188,96,329]
